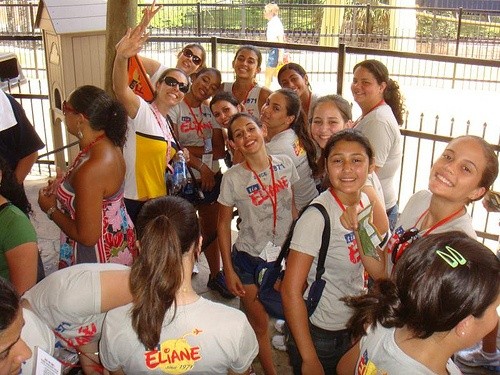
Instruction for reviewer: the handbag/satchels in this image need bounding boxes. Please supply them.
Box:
[257,203,331,321]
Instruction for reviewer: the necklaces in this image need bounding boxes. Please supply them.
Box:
[60,134,105,183]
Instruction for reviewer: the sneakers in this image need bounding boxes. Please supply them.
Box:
[454,346,500,375]
[207,271,237,299]
[274,319,286,333]
[271,335,288,351]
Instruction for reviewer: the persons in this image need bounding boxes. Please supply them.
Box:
[0,278,56,375]
[38,85,137,270]
[262,4,286,89]
[339,134,500,345]
[166,46,325,299]
[0,87,46,285]
[349,60,406,236]
[457,250,500,367]
[0,157,37,306]
[20,263,132,375]
[273,95,399,350]
[282,129,388,375]
[336,231,500,375]
[217,112,300,375]
[99,197,259,375]
[112,26,190,240]
[114,0,212,107]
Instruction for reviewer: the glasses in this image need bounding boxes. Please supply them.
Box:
[160,76,189,93]
[391,227,420,265]
[182,48,201,66]
[62,100,89,121]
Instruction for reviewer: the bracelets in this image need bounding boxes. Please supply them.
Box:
[197,162,203,171]
[47,208,57,219]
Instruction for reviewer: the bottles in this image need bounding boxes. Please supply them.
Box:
[172,150,187,191]
